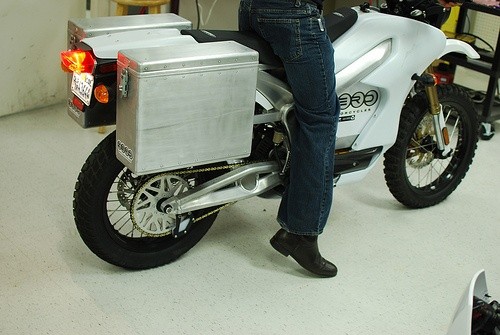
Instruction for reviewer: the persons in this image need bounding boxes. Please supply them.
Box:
[238,0,463,277]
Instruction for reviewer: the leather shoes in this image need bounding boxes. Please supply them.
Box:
[269,228,338,276]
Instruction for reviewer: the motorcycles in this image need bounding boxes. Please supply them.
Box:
[59,0,486,272]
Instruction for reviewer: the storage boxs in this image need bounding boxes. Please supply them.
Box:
[67,12,193,129]
[116,40,259,176]
